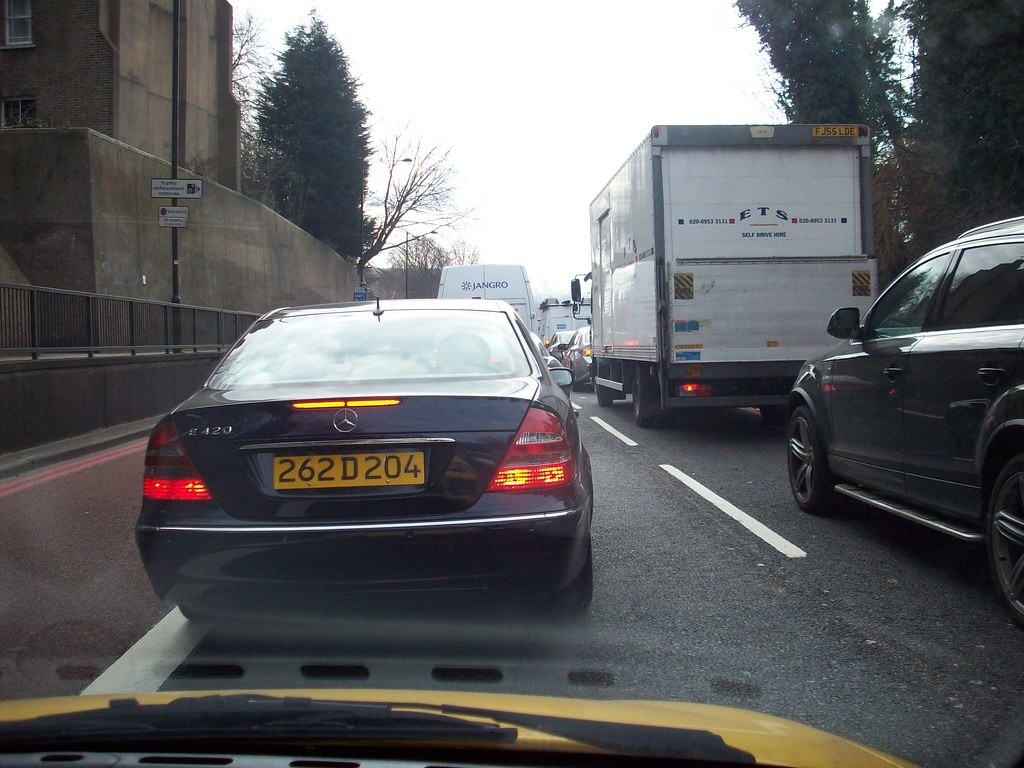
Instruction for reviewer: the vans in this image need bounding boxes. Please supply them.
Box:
[433,262,538,345]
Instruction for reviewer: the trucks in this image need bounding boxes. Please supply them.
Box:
[570,123,883,429]
[538,297,592,343]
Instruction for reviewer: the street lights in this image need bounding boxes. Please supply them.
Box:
[359,157,413,288]
[404,229,438,298]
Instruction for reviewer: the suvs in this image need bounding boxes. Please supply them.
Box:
[782,215,1024,626]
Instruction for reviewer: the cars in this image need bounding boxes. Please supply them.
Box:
[557,326,597,391]
[544,330,577,361]
[136,296,596,625]
